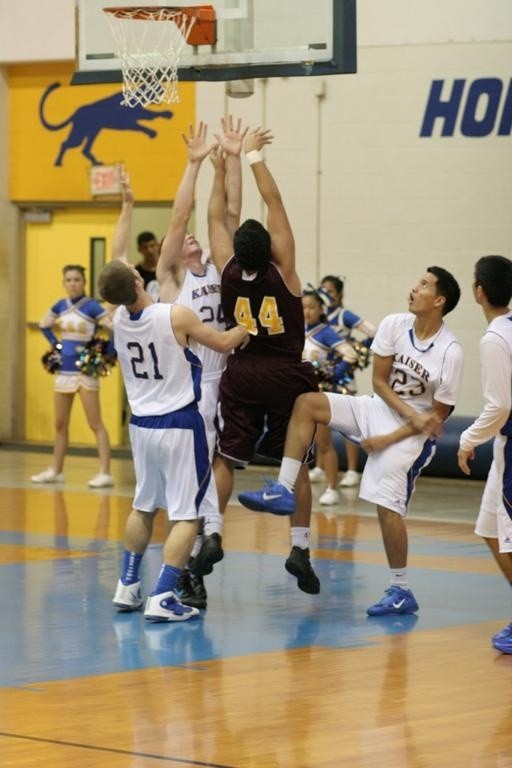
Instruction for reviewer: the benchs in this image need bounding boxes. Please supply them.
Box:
[247,412,492,479]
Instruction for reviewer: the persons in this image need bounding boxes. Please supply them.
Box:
[239,267,465,618]
[459,254,512,653]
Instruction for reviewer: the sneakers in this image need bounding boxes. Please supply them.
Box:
[319,487,342,506]
[236,480,297,517]
[365,584,421,617]
[143,591,201,624]
[489,621,512,657]
[110,576,145,612]
[307,465,325,483]
[30,468,67,485]
[177,532,224,609]
[284,545,321,595]
[87,471,115,489]
[339,469,362,488]
